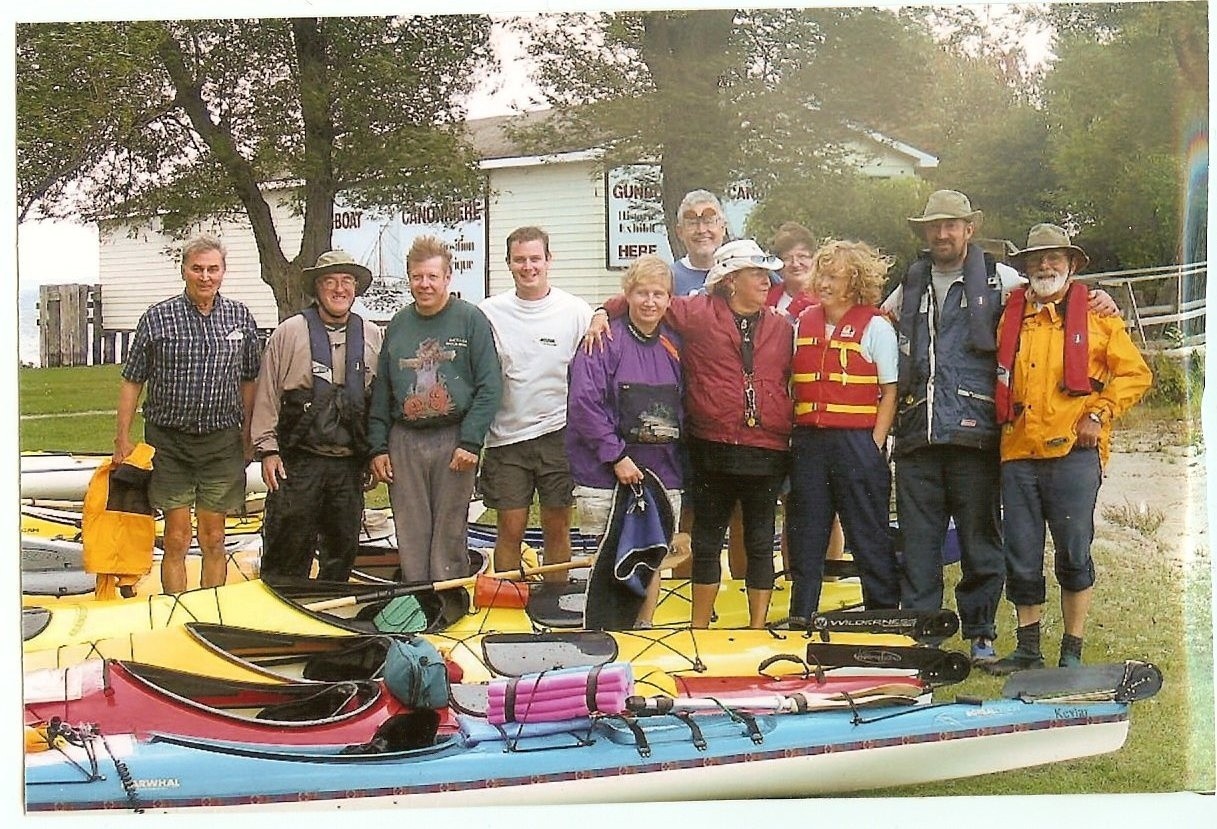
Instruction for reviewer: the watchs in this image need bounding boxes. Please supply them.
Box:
[1085,412,1102,426]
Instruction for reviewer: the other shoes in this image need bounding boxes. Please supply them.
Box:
[982,652,1042,675]
[1059,655,1082,667]
[970,644,996,664]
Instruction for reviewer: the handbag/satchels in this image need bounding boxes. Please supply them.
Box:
[386,638,448,706]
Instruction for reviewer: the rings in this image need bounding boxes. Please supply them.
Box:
[381,472,387,479]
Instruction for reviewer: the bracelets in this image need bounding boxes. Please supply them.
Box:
[590,312,608,319]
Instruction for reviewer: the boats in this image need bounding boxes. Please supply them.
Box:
[23,658,1165,815]
[21,443,973,744]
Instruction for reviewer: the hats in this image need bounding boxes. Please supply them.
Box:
[704,240,784,295]
[907,190,983,241]
[299,250,373,299]
[1007,223,1089,276]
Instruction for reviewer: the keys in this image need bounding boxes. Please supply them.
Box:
[625,498,649,515]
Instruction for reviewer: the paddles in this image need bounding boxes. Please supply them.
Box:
[304,553,595,614]
[625,682,924,714]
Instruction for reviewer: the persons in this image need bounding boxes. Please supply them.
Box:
[251,251,382,583]
[366,238,503,586]
[113,235,262,595]
[578,190,1152,676]
[566,255,691,631]
[476,225,594,584]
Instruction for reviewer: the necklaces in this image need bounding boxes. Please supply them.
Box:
[727,308,762,342]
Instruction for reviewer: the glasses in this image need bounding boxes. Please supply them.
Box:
[720,254,776,263]
[317,279,359,291]
[1024,252,1070,266]
